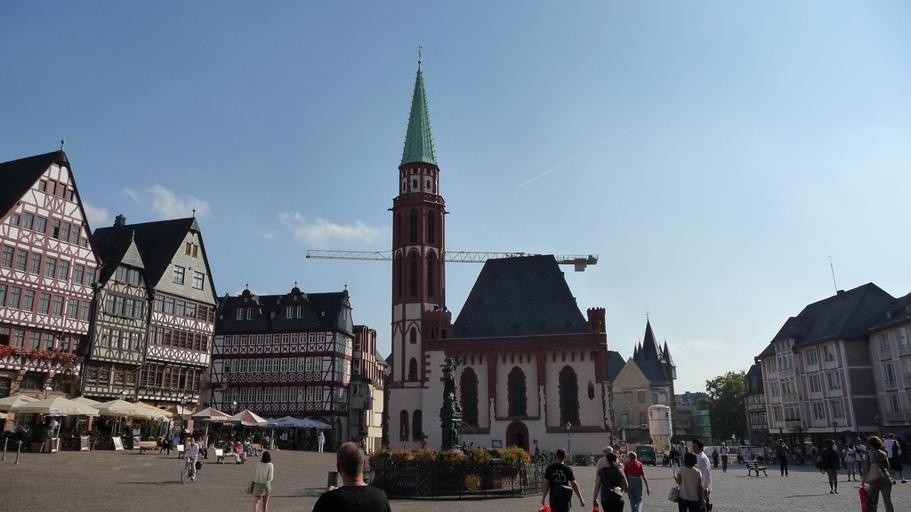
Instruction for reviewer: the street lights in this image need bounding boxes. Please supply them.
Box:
[730,434,736,447]
[228,400,238,452]
[178,394,186,455]
[831,418,840,450]
[565,420,574,466]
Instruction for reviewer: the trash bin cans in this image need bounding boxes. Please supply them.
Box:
[327,472,337,488]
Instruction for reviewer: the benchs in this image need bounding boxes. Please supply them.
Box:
[744,459,769,478]
[139,440,264,465]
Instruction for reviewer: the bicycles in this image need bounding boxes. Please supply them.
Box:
[179,455,200,485]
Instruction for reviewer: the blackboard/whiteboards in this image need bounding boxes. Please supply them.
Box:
[112,437,122,450]
[80,436,90,450]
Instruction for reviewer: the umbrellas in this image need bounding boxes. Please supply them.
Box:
[0,393,174,438]
[163,403,332,450]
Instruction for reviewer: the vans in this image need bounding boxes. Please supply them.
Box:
[634,444,657,465]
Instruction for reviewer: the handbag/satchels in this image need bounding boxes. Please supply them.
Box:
[605,487,624,511]
[815,449,833,470]
[667,487,680,503]
[244,485,254,495]
[859,485,873,511]
[196,461,203,470]
[557,483,573,504]
[867,449,888,472]
[691,501,713,512]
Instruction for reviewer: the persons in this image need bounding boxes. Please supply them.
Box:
[679,440,690,454]
[441,354,465,411]
[720,442,731,472]
[843,443,858,482]
[184,433,270,463]
[1,415,112,452]
[881,433,909,484]
[624,450,651,512]
[592,445,624,480]
[594,452,630,510]
[312,442,391,511]
[737,432,911,478]
[251,450,275,511]
[540,448,586,512]
[317,431,326,454]
[822,439,841,494]
[182,437,200,480]
[670,444,676,467]
[862,435,895,511]
[675,453,707,512]
[712,445,720,467]
[691,437,713,511]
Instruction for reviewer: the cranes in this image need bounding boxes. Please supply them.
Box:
[304,248,601,273]
